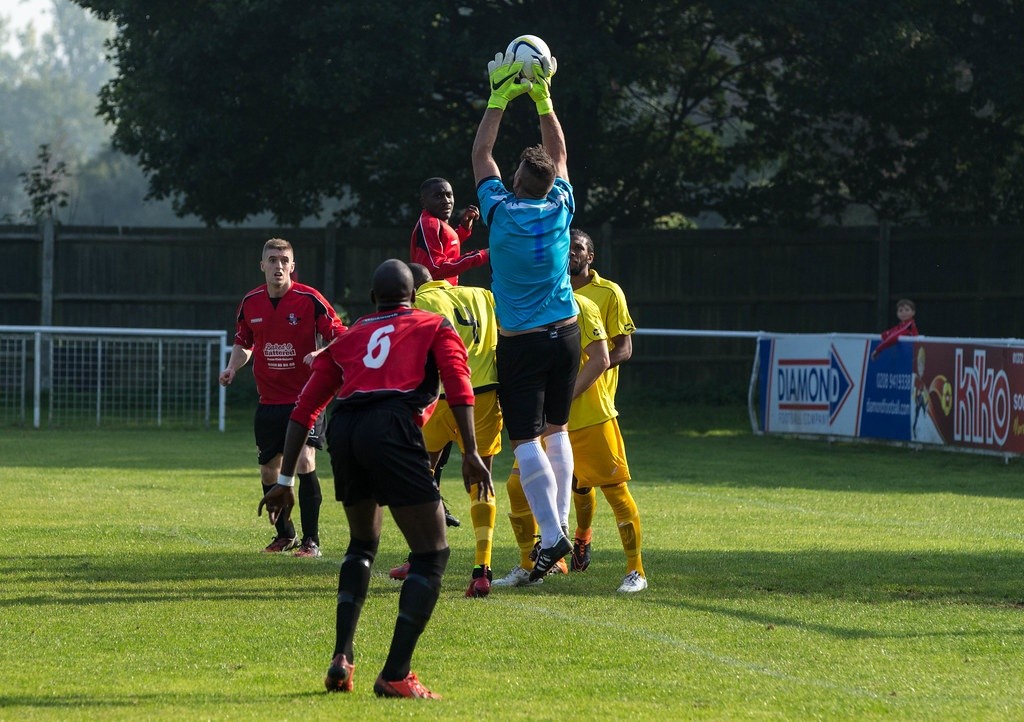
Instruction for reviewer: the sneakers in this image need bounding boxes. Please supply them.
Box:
[466,565,492,597]
[548,558,568,575]
[446,514,462,526]
[528,526,568,561]
[259,532,301,555]
[571,537,592,573]
[615,570,648,593]
[489,566,544,586]
[373,668,443,701]
[529,532,573,583]
[389,553,412,581]
[292,537,323,559]
[325,653,356,693]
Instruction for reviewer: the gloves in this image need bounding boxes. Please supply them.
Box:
[487,52,532,110]
[520,56,557,115]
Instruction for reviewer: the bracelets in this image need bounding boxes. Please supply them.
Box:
[277,474,296,487]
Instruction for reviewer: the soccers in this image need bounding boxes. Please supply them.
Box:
[506,35,551,85]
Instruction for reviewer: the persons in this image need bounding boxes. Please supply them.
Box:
[471,52,581,584]
[410,177,489,528]
[545,229,636,575]
[871,298,919,360]
[389,264,504,598]
[257,259,494,700]
[491,293,648,592]
[219,238,348,557]
